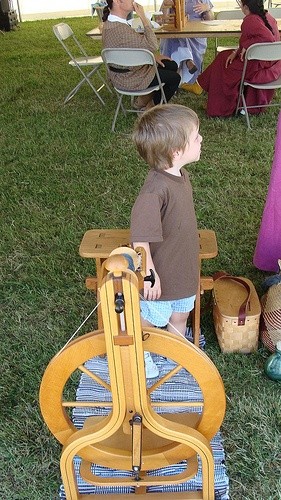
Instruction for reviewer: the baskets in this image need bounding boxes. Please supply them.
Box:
[211,274,261,353]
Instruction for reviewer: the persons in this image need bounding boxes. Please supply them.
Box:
[251,114,281,381]
[179,0,281,118]
[131,104,203,379]
[101,0,181,118]
[157,0,214,85]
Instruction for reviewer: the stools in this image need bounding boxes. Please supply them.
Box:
[79,228,218,346]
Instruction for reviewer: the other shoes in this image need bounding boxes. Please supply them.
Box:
[133,94,152,110]
[135,99,155,118]
[180,81,202,96]
[184,60,197,74]
[142,351,159,379]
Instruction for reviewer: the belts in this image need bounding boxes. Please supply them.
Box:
[107,66,129,73]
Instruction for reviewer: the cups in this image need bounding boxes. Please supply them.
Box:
[153,11,163,26]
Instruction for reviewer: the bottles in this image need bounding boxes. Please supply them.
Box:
[265,341,281,381]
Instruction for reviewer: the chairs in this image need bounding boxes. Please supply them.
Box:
[235,42,281,129]
[215,8,281,54]
[101,48,167,132]
[53,23,110,108]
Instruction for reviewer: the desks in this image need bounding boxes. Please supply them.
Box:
[86,19,281,41]
